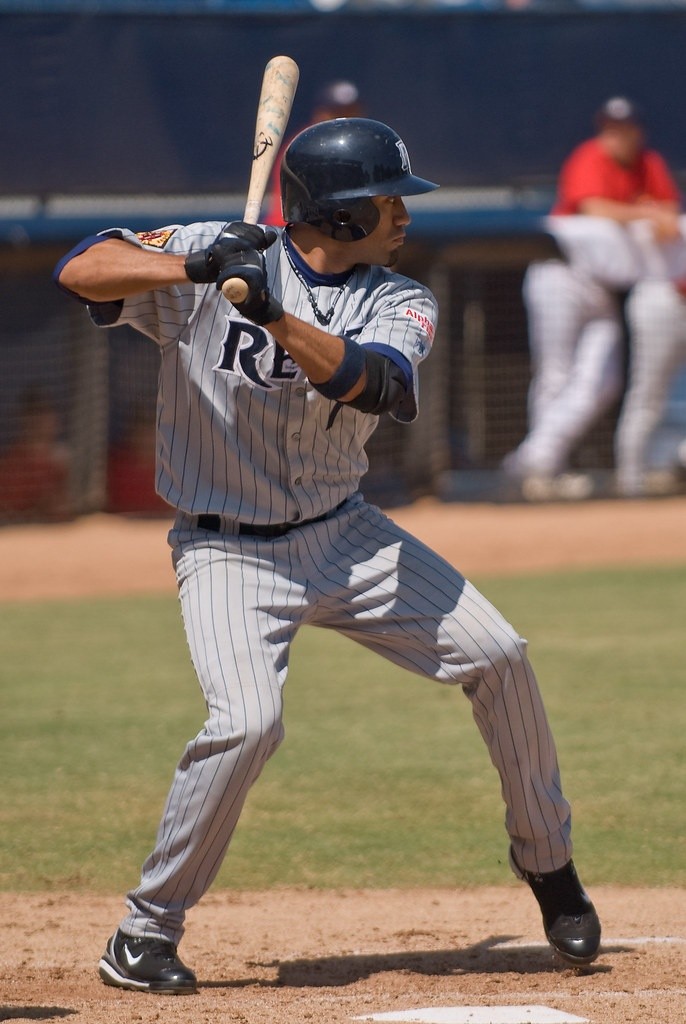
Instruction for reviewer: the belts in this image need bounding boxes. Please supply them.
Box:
[198,498,348,538]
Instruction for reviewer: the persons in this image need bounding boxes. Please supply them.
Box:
[265,80,685,501]
[54,117,603,994]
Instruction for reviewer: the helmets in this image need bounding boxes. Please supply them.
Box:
[281,119,440,242]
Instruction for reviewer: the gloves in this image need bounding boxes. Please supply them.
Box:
[216,249,285,327]
[184,222,277,284]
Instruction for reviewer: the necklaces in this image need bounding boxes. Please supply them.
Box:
[279,228,354,326]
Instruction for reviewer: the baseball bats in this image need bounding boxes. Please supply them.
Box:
[218,52,302,307]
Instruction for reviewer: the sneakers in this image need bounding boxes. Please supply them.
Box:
[508,844,601,966]
[99,927,197,995]
